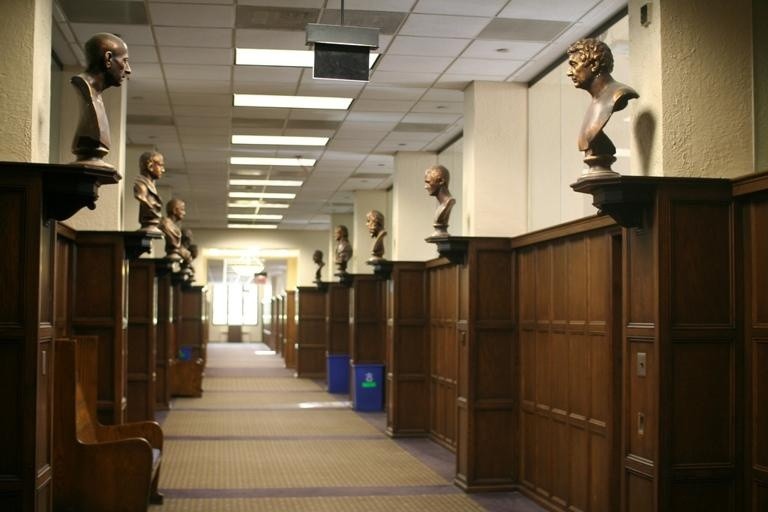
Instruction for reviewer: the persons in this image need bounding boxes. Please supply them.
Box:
[364,211,386,263]
[161,198,186,262]
[334,224,351,275]
[133,152,166,235]
[185,246,198,274]
[312,247,326,284]
[70,33,132,167]
[568,40,641,183]
[423,165,455,238]
[182,228,193,262]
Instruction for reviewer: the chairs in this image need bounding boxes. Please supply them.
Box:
[171,323,205,399]
[55,335,164,512]
